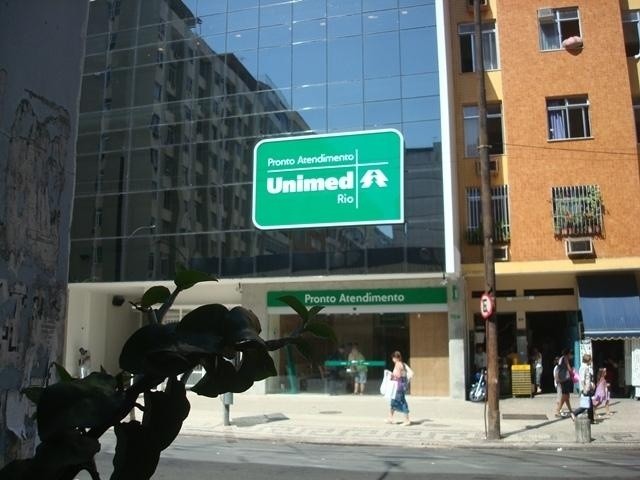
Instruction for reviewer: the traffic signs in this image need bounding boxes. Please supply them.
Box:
[252,128,405,229]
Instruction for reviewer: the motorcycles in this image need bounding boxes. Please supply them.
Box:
[468,367,487,402]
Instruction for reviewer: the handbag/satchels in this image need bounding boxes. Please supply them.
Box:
[580,393,590,409]
[582,382,596,396]
[557,355,569,380]
[402,364,414,383]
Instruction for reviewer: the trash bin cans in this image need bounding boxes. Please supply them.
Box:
[576,417,591,443]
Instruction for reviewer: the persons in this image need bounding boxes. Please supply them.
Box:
[474,343,543,393]
[328,341,368,395]
[383,351,411,426]
[553,347,613,424]
